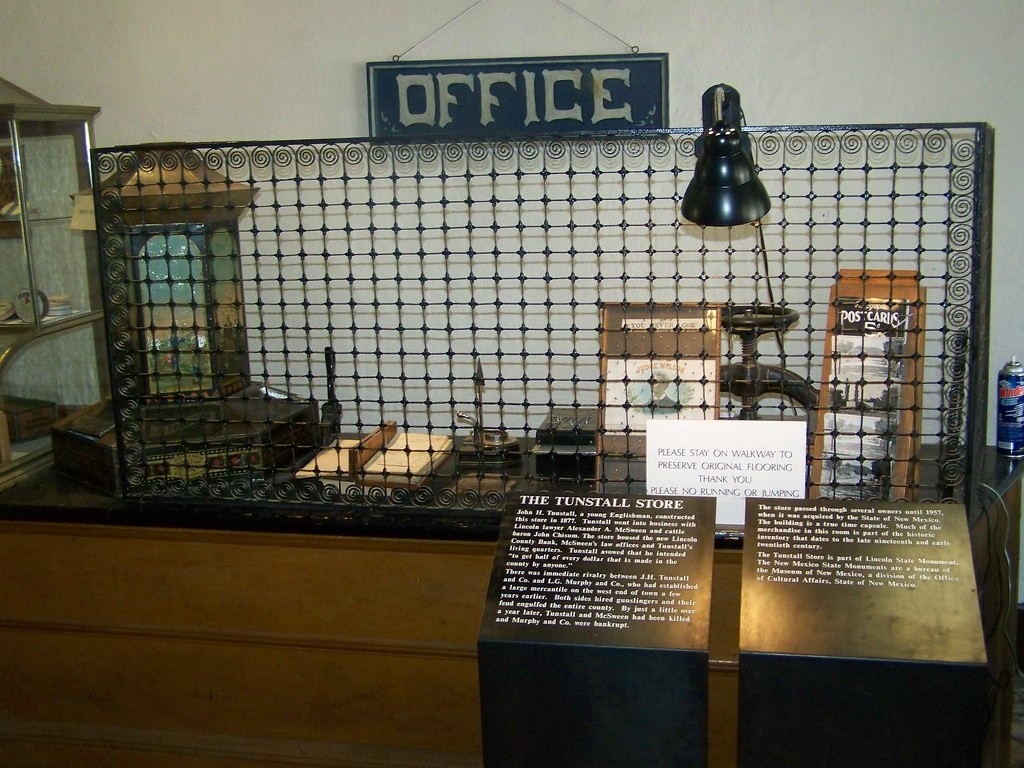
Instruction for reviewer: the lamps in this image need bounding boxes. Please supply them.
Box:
[680,81,770,229]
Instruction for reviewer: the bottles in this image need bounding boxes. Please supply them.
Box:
[996,355,1024,458]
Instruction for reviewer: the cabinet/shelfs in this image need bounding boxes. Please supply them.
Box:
[0,77,113,493]
